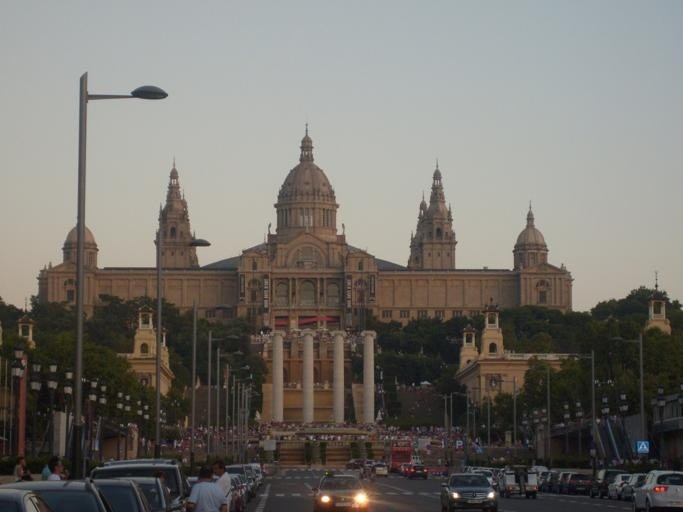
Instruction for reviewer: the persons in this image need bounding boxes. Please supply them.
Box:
[157,326,534,467]
[213,459,232,512]
[13,456,71,482]
[186,467,228,512]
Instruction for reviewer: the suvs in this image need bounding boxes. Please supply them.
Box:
[588,469,628,499]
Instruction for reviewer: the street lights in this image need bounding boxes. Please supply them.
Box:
[437,350,600,466]
[677,381,682,420]
[617,389,631,465]
[187,297,262,463]
[71,68,171,476]
[610,330,646,458]
[602,390,610,440]
[376,364,388,418]
[652,385,668,459]
[152,198,212,455]
[6,343,173,459]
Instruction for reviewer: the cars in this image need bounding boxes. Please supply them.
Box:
[279,417,480,450]
[0,457,265,512]
[618,474,646,502]
[630,470,682,512]
[440,472,497,512]
[310,470,370,512]
[606,474,630,499]
[345,439,592,498]
